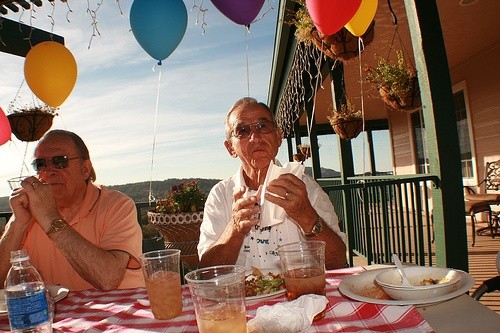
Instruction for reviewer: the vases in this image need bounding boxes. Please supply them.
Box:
[148,211,204,271]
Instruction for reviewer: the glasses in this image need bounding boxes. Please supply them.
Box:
[32,156,86,171]
[231,121,276,139]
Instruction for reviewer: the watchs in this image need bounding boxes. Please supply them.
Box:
[46,217,66,237]
[303,217,323,238]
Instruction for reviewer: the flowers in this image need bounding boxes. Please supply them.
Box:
[155,180,207,212]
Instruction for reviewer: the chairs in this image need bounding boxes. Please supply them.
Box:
[431,160,500,247]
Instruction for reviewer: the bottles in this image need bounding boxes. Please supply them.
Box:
[4,248,54,333]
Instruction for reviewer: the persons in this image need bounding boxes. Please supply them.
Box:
[197,97,348,279]
[0,129,145,291]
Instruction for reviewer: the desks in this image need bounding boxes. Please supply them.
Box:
[0,262,500,333]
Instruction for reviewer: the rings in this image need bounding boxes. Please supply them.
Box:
[31,181,36,186]
[284,191,289,197]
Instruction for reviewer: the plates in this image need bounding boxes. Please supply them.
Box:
[245,267,286,307]
[337,265,475,306]
[0,284,69,318]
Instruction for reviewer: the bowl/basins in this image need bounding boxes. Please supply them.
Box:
[374,267,462,300]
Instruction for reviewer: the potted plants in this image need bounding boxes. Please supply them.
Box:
[7,106,59,142]
[328,99,365,140]
[293,153,306,162]
[296,143,320,159]
[363,49,423,113]
[283,0,374,63]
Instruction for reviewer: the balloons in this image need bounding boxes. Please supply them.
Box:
[24,41,77,108]
[343,0,379,38]
[306,0,362,40]
[210,0,265,30]
[0,107,12,146]
[129,0,188,65]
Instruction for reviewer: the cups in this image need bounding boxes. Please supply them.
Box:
[276,240,327,322]
[184,264,247,333]
[137,249,181,320]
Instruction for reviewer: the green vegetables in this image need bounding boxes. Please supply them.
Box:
[254,277,285,295]
